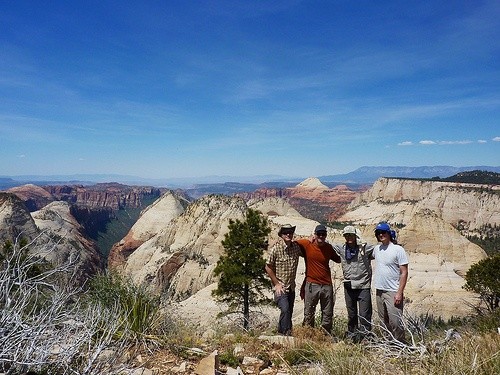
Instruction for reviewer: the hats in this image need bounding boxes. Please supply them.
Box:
[375,224,396,239]
[278,224,296,237]
[342,225,360,239]
[315,225,327,232]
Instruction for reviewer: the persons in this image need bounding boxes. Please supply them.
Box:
[265,223,304,337]
[371,223,409,344]
[307,225,407,337]
[268,224,341,336]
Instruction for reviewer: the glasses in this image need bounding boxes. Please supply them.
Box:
[376,230,387,234]
